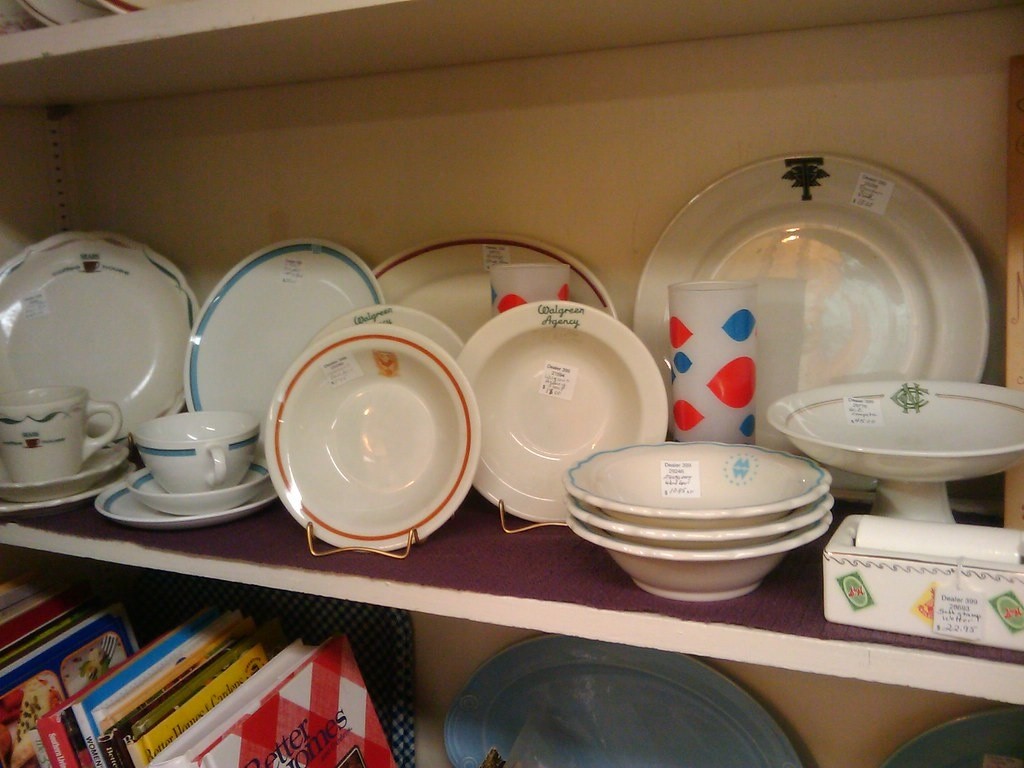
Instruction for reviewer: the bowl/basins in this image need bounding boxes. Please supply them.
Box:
[563,441,834,603]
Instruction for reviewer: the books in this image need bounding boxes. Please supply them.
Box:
[0,554,421,768]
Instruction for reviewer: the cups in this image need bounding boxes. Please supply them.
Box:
[669,280,759,445]
[491,264,570,317]
[133,410,259,495]
[0,385,122,482]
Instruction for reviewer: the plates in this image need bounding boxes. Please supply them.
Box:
[0,441,129,502]
[265,324,480,553]
[879,706,1024,768]
[372,234,616,346]
[306,306,465,359]
[633,152,988,490]
[94,483,278,530]
[0,230,199,447]
[444,634,802,768]
[767,380,1024,482]
[457,300,669,524]
[124,456,270,514]
[0,459,133,517]
[184,238,383,461]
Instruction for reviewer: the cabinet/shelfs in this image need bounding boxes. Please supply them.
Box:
[0,0,1024,705]
